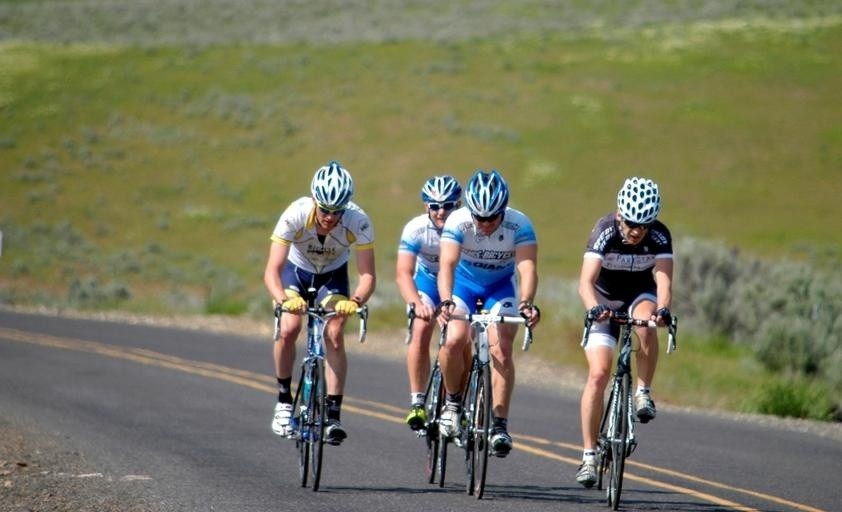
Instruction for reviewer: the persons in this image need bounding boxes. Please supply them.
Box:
[396,174,469,449]
[575,177,673,484]
[435,168,537,456]
[271,161,376,445]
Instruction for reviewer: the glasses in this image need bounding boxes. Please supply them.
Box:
[428,202,457,211]
[621,217,655,230]
[316,202,344,216]
[471,211,503,223]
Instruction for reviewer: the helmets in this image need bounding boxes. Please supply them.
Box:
[615,175,664,225]
[310,159,355,208]
[420,174,464,203]
[463,168,510,218]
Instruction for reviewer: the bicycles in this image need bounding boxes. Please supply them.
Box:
[403,301,453,489]
[428,298,534,502]
[270,298,370,493]
[578,309,679,512]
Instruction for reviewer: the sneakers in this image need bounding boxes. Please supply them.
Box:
[437,401,464,438]
[488,427,513,459]
[405,402,427,431]
[270,399,297,439]
[574,459,600,485]
[321,417,349,446]
[633,391,657,421]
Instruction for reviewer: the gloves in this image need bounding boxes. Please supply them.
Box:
[519,303,541,325]
[649,306,673,327]
[586,304,615,323]
[333,298,360,316]
[282,295,308,311]
[432,298,457,319]
